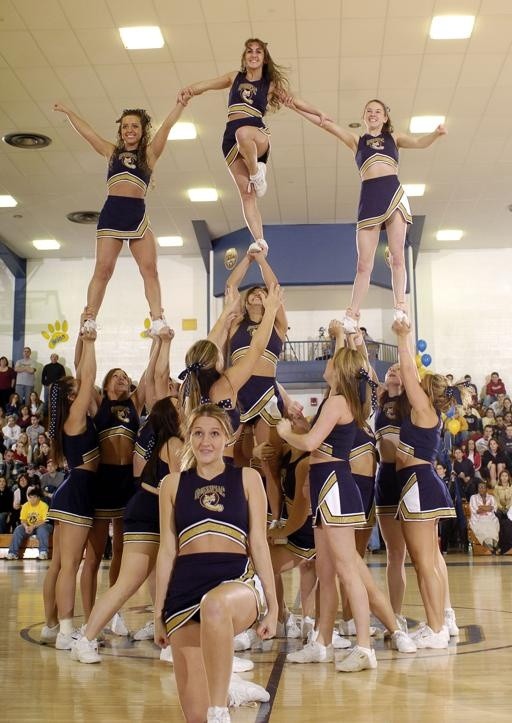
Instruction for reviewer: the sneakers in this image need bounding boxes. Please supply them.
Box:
[36,551,49,560]
[232,655,254,673]
[38,611,174,664]
[286,640,335,663]
[228,671,271,708]
[394,309,410,328]
[150,319,169,336]
[5,552,20,560]
[340,316,359,334]
[248,238,268,253]
[335,645,378,672]
[207,706,231,723]
[234,609,459,653]
[249,161,267,198]
[81,319,97,334]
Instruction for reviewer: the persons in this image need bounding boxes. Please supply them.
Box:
[151,401,281,723]
[52,85,191,335]
[222,248,288,528]
[1,346,66,560]
[244,309,459,670]
[43,285,285,663]
[279,93,450,334]
[440,369,512,553]
[178,34,336,252]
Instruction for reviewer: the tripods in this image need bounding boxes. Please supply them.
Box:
[310,334,332,361]
[278,333,299,362]
